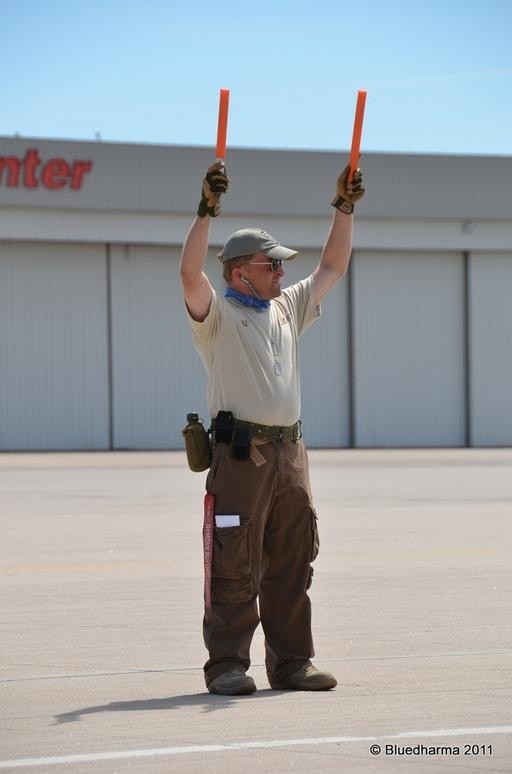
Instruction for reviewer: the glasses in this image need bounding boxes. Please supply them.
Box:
[248,258,284,272]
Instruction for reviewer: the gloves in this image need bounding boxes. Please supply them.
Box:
[196,161,230,218]
[331,152,365,214]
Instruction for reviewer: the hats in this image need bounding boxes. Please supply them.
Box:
[217,228,298,265]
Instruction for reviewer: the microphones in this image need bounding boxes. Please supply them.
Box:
[240,275,261,302]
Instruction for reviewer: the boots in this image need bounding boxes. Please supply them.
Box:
[270,659,338,691]
[208,668,257,696]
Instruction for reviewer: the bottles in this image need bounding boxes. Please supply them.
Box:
[182,411,213,473]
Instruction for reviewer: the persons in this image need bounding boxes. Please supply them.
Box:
[179,153,365,696]
